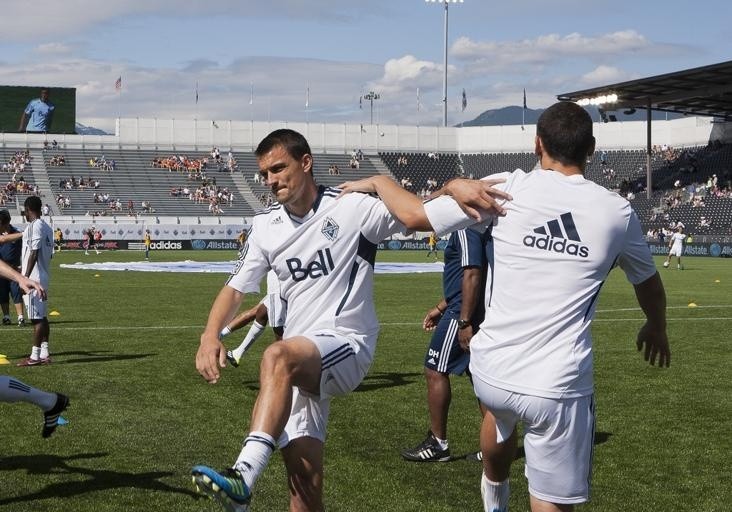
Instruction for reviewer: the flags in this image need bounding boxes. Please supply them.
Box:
[115,77,120,92]
[462,90,468,112]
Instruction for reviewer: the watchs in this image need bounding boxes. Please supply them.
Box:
[456,318,470,328]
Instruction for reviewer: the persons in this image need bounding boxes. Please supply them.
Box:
[218,295,268,342]
[226,269,290,369]
[402,228,490,463]
[0,258,71,438]
[18,86,54,134]
[2,136,732,270]
[191,128,514,511]
[0,210,27,326]
[15,197,54,366]
[335,99,671,511]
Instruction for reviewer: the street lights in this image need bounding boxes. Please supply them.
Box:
[363,91,381,125]
[424,0,465,126]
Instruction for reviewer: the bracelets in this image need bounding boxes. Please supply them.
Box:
[435,304,445,315]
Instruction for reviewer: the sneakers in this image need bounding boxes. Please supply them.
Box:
[399,429,451,463]
[39,355,52,365]
[226,350,240,369]
[2,317,11,325]
[15,357,41,367]
[464,449,484,463]
[84,251,102,255]
[190,464,254,512]
[42,392,71,439]
[18,318,27,327]
